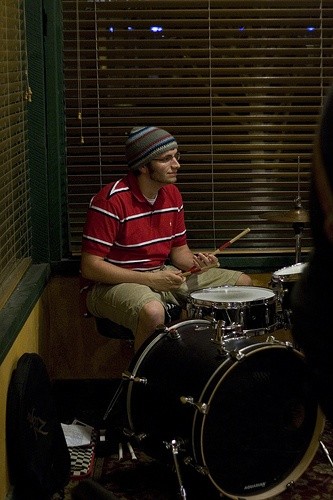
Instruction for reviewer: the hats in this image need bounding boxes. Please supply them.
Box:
[125,126,179,172]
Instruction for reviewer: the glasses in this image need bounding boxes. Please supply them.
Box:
[154,151,180,163]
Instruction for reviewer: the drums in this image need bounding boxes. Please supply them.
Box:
[124,318,327,499]
[271,263,311,329]
[187,285,278,336]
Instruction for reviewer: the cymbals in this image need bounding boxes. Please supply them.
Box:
[259,209,310,222]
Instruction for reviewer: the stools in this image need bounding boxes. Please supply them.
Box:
[95,304,184,357]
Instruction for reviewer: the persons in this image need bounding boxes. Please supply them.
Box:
[79,126,253,353]
[292,87,333,425]
